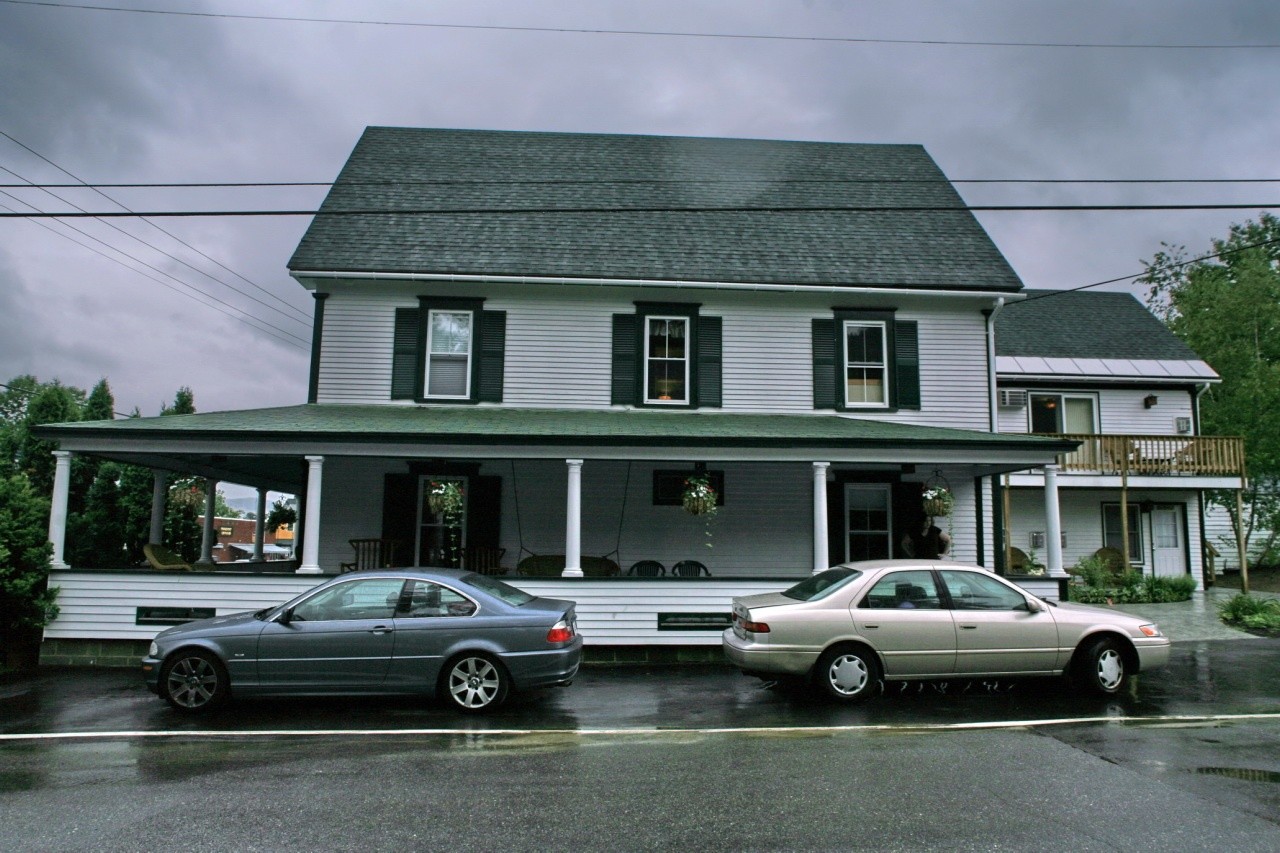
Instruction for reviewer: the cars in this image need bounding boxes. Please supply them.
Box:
[720,558,1172,708]
[139,566,585,721]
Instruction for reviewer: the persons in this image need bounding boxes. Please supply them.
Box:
[902,513,951,561]
[897,586,927,608]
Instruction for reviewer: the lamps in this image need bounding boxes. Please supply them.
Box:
[1144,393,1157,409]
[1061,531,1067,547]
[1029,531,1044,547]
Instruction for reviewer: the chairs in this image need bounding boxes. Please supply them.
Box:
[466,547,509,577]
[627,559,667,576]
[339,537,394,573]
[672,560,711,577]
[144,544,199,571]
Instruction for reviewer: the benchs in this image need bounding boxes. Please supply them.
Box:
[517,554,621,576]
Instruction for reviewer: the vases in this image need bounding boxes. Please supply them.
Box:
[925,500,947,516]
[688,500,704,515]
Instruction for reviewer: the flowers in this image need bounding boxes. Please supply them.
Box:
[426,480,467,562]
[683,473,718,545]
[921,488,955,516]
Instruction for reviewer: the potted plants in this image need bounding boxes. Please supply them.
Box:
[268,502,297,532]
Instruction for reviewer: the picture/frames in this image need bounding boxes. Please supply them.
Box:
[430,497,446,511]
